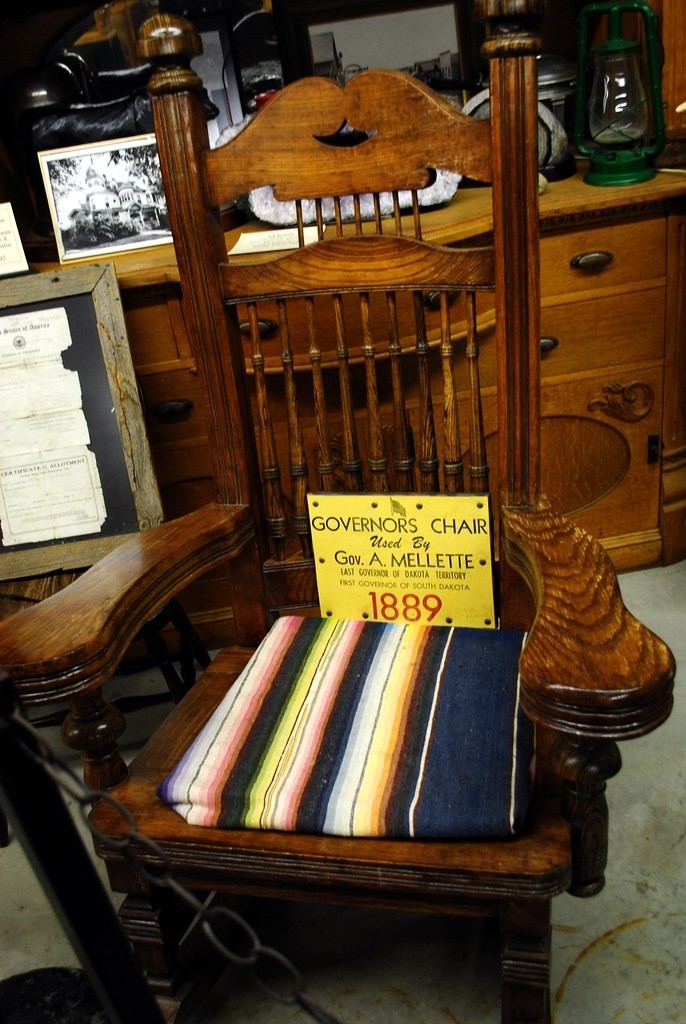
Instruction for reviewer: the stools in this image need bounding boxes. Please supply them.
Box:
[0,583,212,848]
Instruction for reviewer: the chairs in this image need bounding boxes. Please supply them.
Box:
[0,19,679,1024]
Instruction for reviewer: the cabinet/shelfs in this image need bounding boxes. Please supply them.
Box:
[32,177,685,667]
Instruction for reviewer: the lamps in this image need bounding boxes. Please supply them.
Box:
[568,2,666,188]
